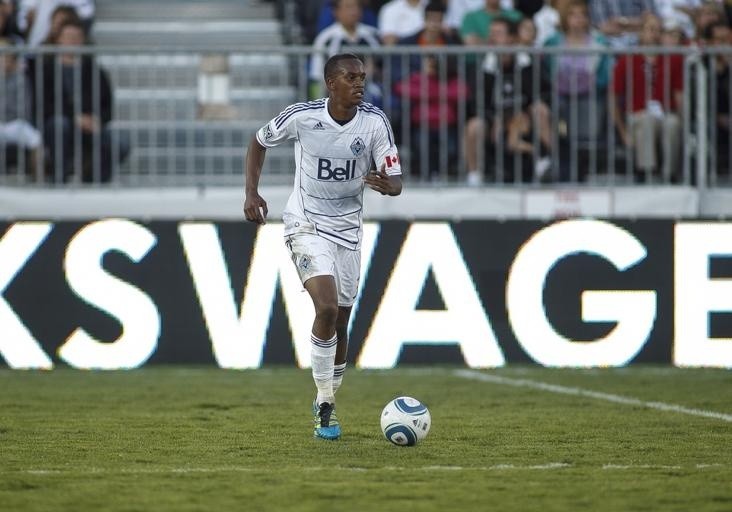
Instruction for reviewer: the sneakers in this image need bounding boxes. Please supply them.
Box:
[312,397,342,440]
[535,154,550,177]
[468,170,485,185]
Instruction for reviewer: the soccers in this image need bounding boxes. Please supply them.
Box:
[380,397,431,446]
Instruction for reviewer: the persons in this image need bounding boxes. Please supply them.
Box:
[309,0,731,185]
[245,53,403,440]
[0,1,130,182]
